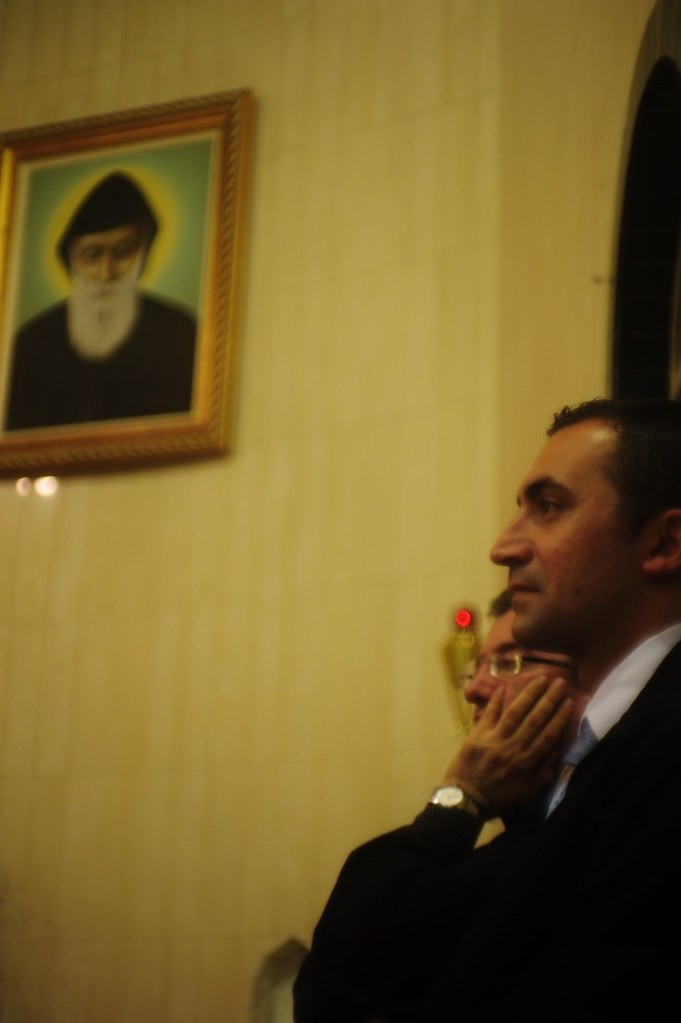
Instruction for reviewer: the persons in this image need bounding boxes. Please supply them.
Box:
[294,590,606,1021]
[420,401,681,1022]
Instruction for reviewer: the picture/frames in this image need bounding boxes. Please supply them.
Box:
[0,89,262,481]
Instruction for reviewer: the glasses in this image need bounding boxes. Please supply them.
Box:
[462,654,575,679]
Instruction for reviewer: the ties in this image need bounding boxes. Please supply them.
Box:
[545,716,600,818]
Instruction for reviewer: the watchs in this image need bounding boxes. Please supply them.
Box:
[427,785,483,820]
